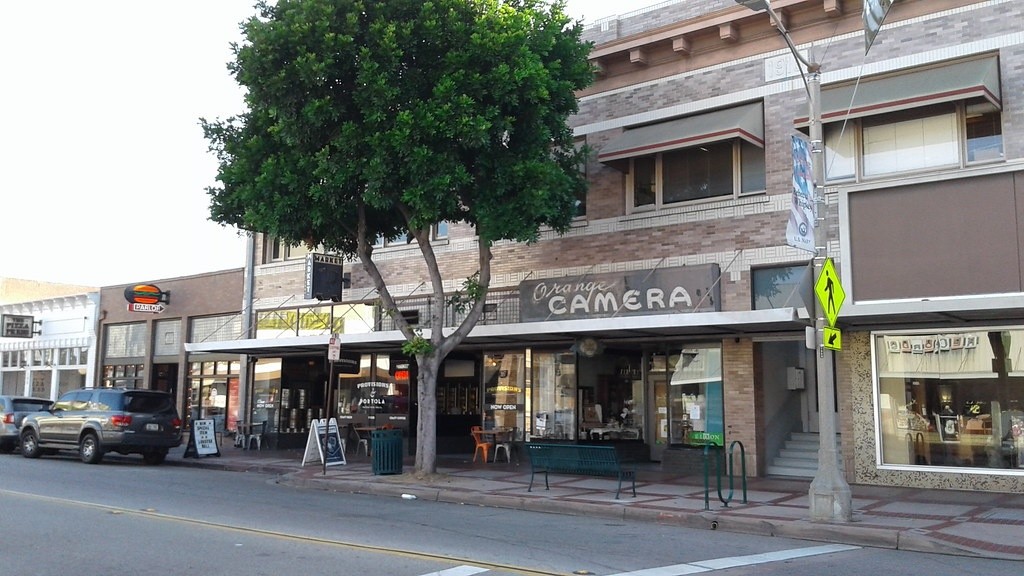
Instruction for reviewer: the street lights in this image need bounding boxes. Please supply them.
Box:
[734,0,854,524]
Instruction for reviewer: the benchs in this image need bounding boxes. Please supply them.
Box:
[523,442,638,500]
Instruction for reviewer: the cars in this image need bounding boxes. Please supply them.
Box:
[0,395,54,452]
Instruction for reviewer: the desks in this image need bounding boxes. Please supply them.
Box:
[239,423,263,435]
[475,430,512,461]
[355,425,400,440]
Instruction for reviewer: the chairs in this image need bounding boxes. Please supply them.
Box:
[382,423,394,430]
[493,426,520,463]
[470,426,494,463]
[247,421,269,451]
[352,423,370,456]
[234,421,246,448]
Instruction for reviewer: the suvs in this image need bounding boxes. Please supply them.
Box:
[17,386,185,465]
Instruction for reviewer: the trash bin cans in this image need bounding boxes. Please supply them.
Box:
[370,429,403,475]
